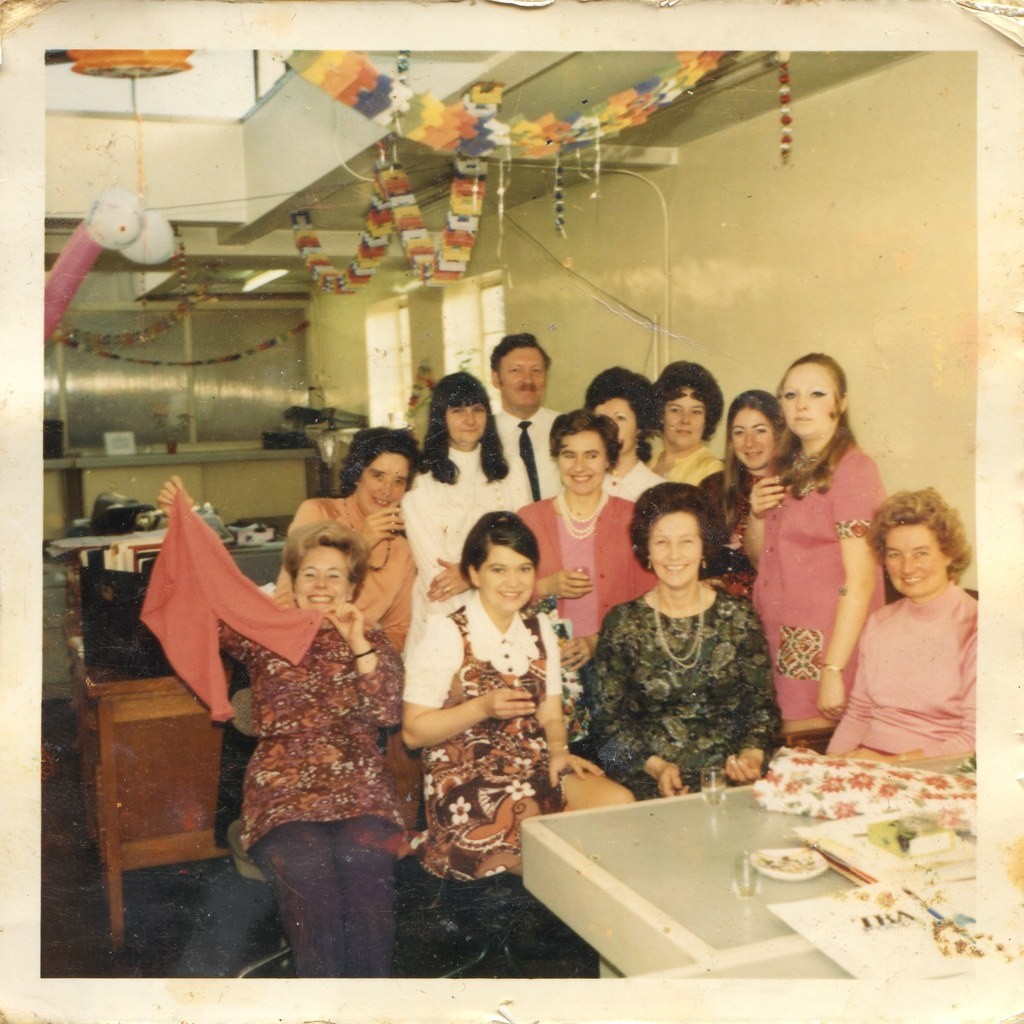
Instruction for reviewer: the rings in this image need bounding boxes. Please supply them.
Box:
[443,585,450,591]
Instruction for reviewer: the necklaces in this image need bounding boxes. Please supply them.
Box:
[344,498,390,571]
[558,488,607,538]
[655,582,704,669]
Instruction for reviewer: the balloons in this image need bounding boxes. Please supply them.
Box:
[45,185,175,345]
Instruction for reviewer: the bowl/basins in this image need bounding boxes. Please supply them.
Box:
[263,431,308,449]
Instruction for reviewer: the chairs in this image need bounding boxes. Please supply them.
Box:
[226,688,539,979]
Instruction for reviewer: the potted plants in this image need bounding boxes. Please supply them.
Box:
[152,413,194,454]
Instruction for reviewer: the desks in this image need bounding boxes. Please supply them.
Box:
[43,449,426,953]
[520,751,977,979]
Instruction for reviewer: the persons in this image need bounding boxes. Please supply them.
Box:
[644,360,726,487]
[590,482,782,801]
[273,426,420,659]
[821,487,978,765]
[491,333,563,513]
[400,510,637,978]
[698,390,787,602]
[744,353,906,755]
[515,410,659,673]
[583,367,665,503]
[156,475,405,978]
[401,372,530,671]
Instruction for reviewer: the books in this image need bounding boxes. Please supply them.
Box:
[792,811,977,888]
[78,537,165,574]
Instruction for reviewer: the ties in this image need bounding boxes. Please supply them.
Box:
[518,420,540,502]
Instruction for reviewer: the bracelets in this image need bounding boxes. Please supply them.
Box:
[823,664,846,673]
[547,736,569,750]
[898,753,909,762]
[352,647,376,659]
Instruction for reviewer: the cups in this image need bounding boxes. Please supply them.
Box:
[572,567,590,589]
[731,850,760,897]
[513,678,541,709]
[700,766,725,804]
[766,476,784,510]
[383,504,402,535]
[43,420,64,459]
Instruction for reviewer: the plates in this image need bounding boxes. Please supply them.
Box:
[765,878,980,982]
[750,849,828,879]
